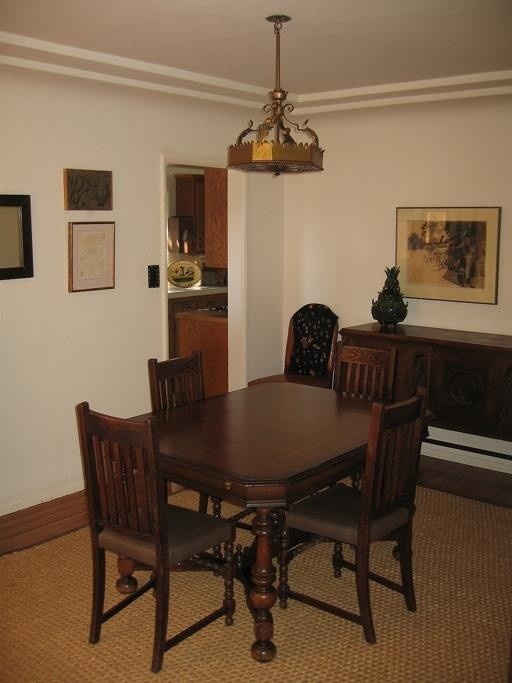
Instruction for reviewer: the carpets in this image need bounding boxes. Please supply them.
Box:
[0,479,511,682]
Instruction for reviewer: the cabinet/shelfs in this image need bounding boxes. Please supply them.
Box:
[175,173,204,255]
[173,305,228,397]
[203,166,228,269]
[339,319,512,447]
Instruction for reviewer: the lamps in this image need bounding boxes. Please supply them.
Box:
[228,12,325,177]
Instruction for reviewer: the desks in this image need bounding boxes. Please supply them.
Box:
[116,373,437,663]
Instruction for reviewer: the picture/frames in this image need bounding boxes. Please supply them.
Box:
[395,205,502,305]
[0,193,35,280]
[61,166,114,212]
[67,219,117,293]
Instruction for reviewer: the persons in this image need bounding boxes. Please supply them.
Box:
[448,237,479,289]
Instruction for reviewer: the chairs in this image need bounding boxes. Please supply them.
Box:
[320,344,401,489]
[244,303,341,387]
[67,398,233,673]
[274,384,434,648]
[143,346,223,574]
